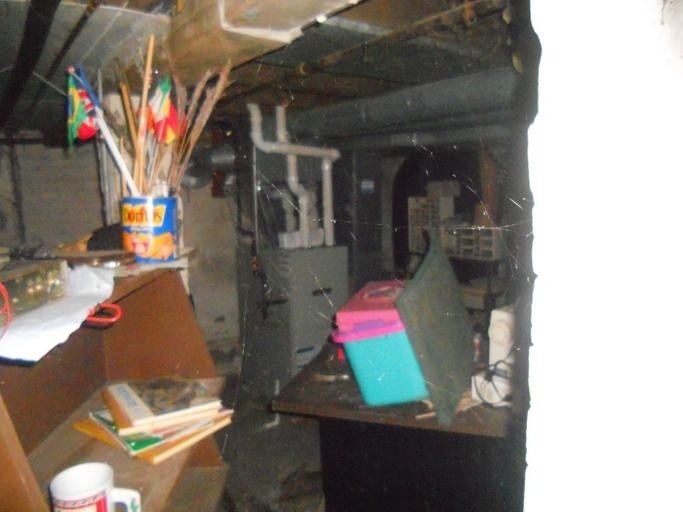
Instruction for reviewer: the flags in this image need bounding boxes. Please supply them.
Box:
[134,72,189,147]
[65,63,100,156]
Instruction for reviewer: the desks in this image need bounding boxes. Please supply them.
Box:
[270,339,506,511]
[1,268,230,512]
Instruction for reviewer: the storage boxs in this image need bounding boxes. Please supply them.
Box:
[331,281,429,408]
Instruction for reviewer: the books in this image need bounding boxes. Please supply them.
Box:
[67,373,235,464]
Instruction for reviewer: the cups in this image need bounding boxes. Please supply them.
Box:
[48,461,143,512]
